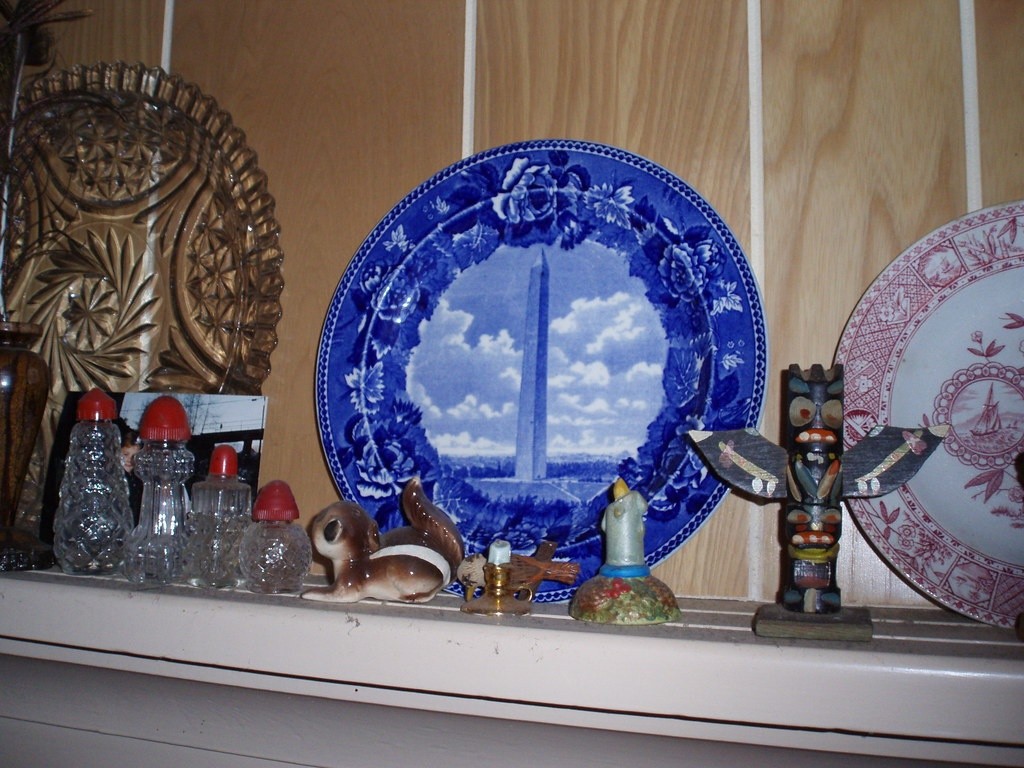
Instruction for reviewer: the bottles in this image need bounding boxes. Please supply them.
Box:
[0,321,52,550]
[52,388,134,576]
[180,444,252,590]
[120,396,198,582]
[237,479,312,596]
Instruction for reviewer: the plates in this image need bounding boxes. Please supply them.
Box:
[313,138,769,607]
[831,200,1023,629]
